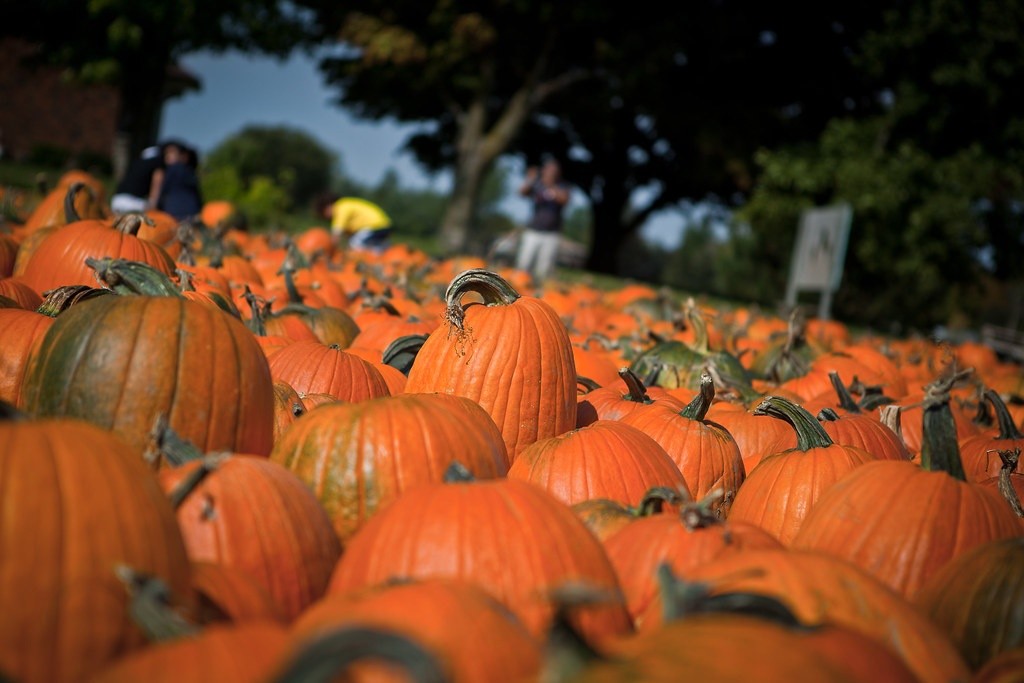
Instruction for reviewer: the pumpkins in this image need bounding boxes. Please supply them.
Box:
[0,170,1024,683]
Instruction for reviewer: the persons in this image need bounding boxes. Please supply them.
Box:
[517,160,570,276]
[164,141,203,217]
[318,193,393,246]
[111,143,162,212]
